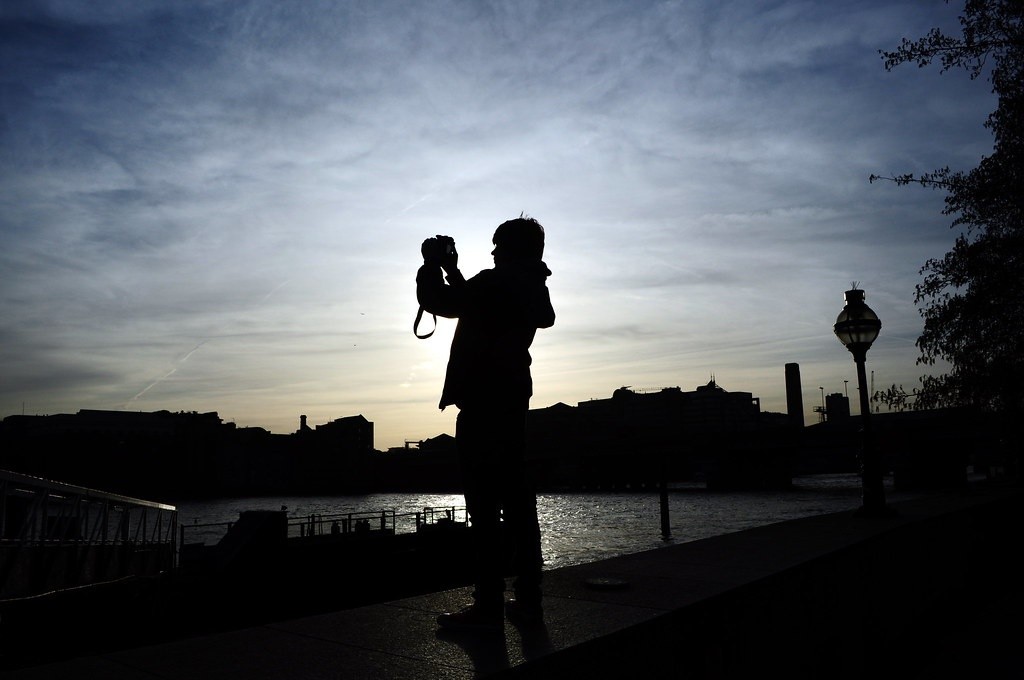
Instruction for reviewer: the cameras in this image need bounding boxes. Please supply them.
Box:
[430,235,455,260]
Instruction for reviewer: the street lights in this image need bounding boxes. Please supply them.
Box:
[833,280,886,508]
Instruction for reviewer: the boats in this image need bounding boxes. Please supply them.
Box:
[1,467,177,611]
[180,506,473,588]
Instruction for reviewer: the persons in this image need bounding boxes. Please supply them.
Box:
[415,218,554,632]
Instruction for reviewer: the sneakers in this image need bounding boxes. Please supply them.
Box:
[505,599,544,625]
[437,602,504,634]
